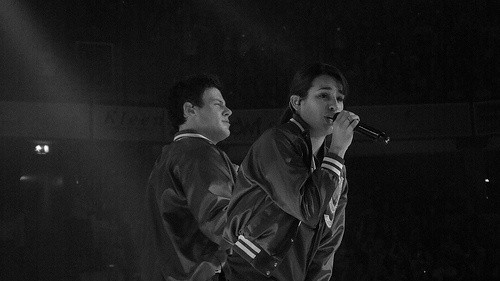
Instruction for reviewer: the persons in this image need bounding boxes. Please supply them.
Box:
[145,77,243,281]
[218,61,360,281]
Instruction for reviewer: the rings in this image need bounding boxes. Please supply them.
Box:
[345,118,350,123]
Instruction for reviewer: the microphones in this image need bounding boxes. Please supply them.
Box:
[332,112,390,144]
